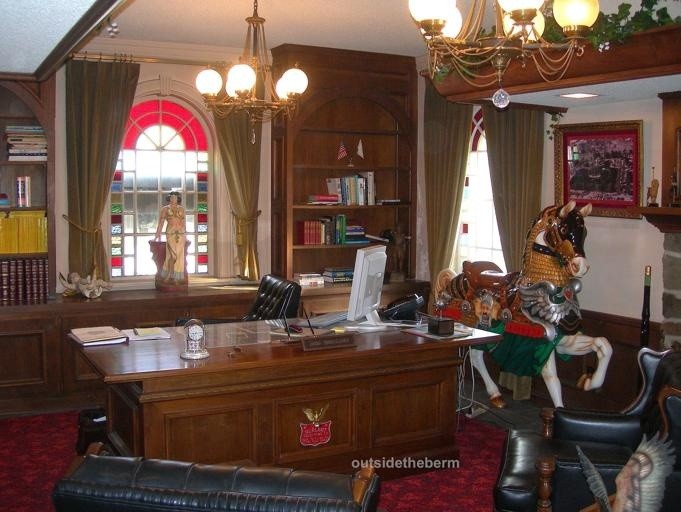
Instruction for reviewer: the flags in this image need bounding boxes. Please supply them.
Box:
[337,140,347,162]
[356,139,366,159]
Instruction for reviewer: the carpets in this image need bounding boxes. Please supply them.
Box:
[2,411,509,512]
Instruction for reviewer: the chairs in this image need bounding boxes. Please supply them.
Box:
[175,274,302,327]
[53,443,381,512]
[493,348,680,512]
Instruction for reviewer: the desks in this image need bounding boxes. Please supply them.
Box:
[67,314,503,480]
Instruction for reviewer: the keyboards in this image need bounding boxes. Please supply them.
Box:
[297,310,349,329]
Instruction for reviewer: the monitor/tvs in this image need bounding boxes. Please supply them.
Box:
[342,244,388,330]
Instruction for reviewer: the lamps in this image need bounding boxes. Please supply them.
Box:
[408,0,599,108]
[195,0,309,145]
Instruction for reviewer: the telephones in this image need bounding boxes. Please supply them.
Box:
[383,294,424,320]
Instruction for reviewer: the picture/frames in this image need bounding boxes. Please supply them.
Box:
[553,120,644,219]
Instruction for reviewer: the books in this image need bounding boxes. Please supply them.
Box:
[0,122,49,306]
[121,327,172,340]
[68,325,130,346]
[294,170,411,290]
[133,326,162,337]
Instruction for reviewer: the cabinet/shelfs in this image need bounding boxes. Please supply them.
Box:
[271,44,416,283]
[0,71,57,310]
[0,283,433,413]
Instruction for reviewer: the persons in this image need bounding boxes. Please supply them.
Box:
[155,191,188,289]
[428,199,615,418]
[564,139,633,204]
[56,268,116,300]
